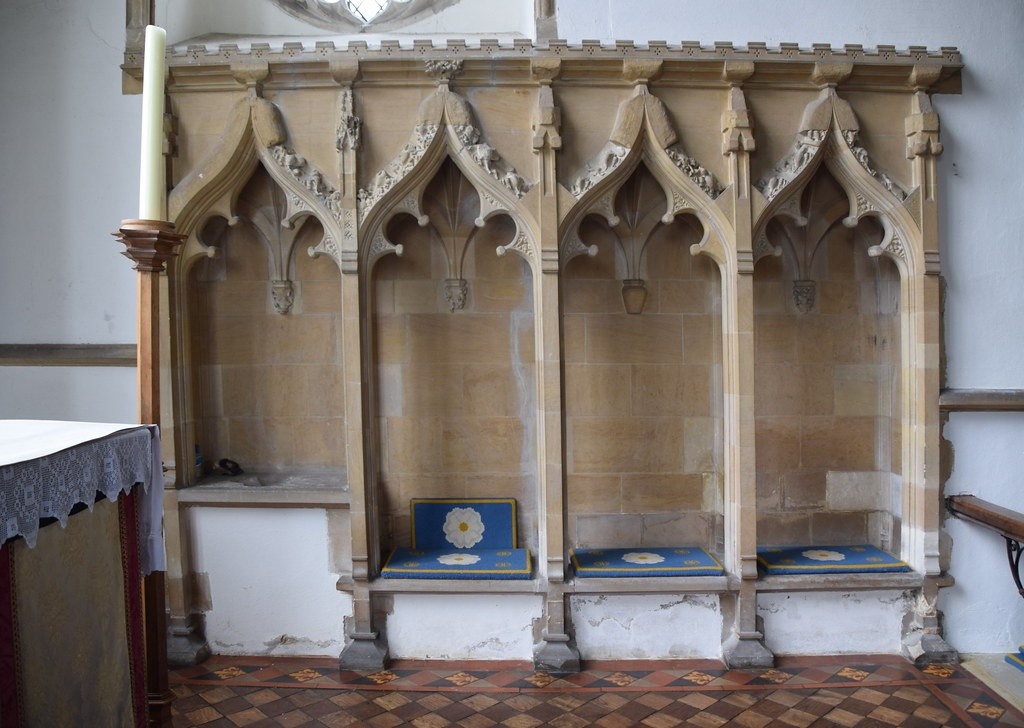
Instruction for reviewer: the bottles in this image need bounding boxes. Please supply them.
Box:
[195,445,203,479]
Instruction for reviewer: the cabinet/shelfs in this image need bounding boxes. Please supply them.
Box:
[1,421,175,727]
